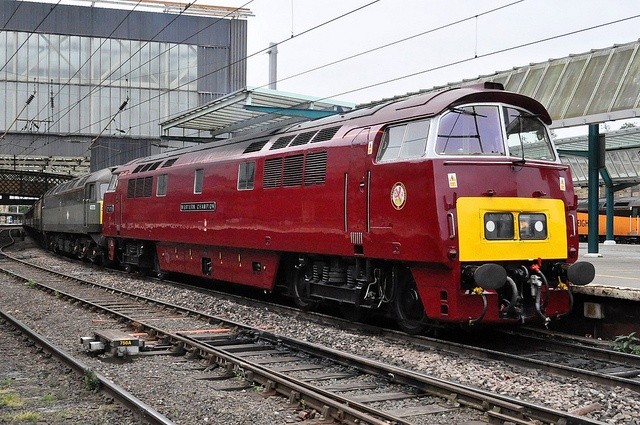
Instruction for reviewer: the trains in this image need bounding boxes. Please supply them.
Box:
[22,81,595,337]
[574,196,640,244]
